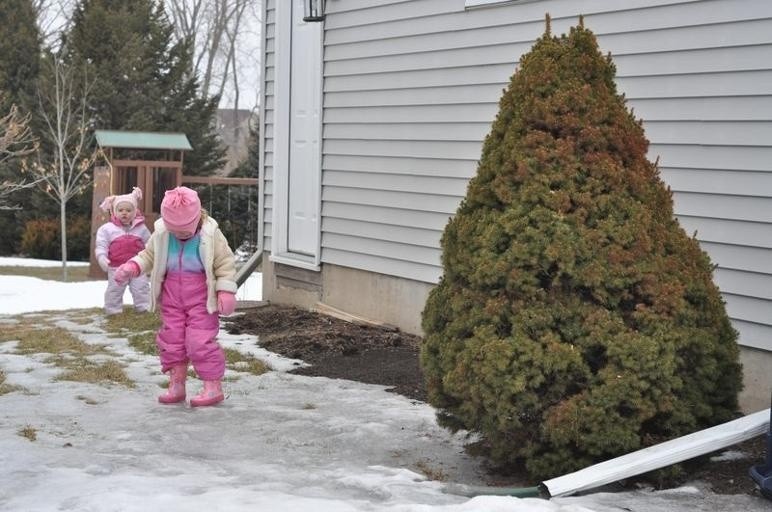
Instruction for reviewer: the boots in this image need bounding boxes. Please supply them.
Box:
[159,365,187,404]
[189,380,224,407]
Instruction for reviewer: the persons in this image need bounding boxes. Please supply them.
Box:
[95,187,151,314]
[114,186,237,406]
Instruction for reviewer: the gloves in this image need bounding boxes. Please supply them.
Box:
[114,262,139,286]
[217,292,235,315]
[98,255,111,272]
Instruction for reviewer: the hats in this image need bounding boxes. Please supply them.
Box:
[160,186,201,236]
[100,186,142,222]
